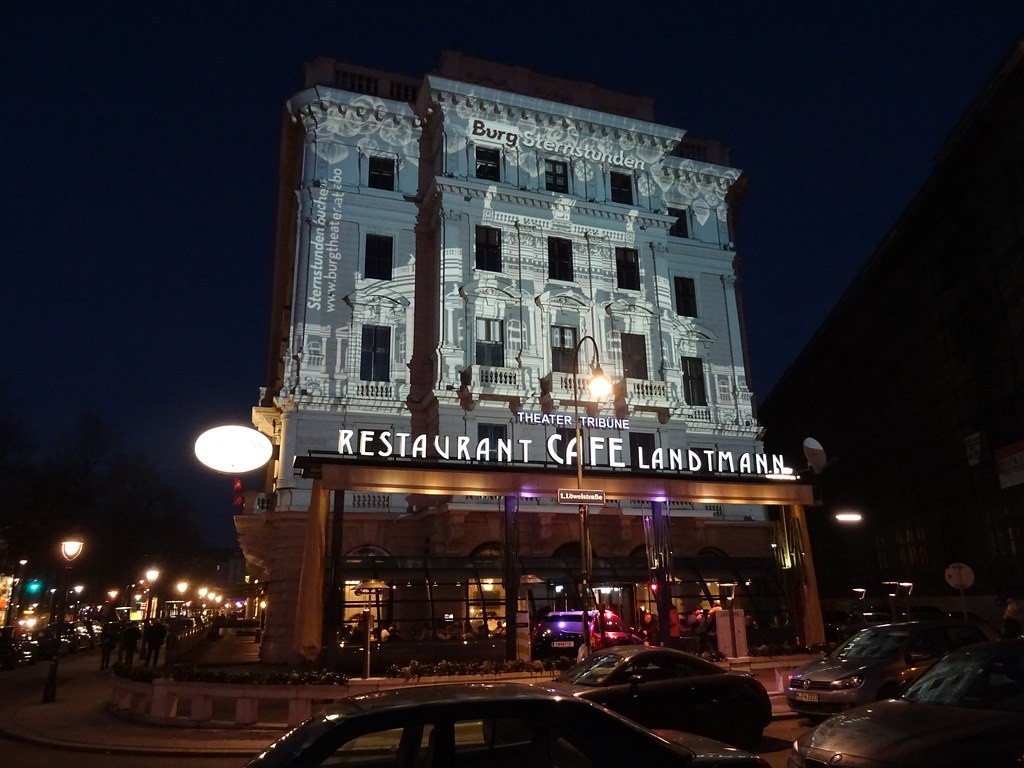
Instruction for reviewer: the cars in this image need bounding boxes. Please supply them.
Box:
[821,606,952,633]
[241,686,768,768]
[480,645,772,749]
[788,637,1024,768]
[786,620,990,723]
[46,619,106,654]
[0,626,39,668]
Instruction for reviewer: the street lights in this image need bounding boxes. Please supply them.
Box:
[571,335,616,656]
[42,532,85,705]
[177,580,187,617]
[352,579,393,679]
[72,583,85,634]
[4,558,27,624]
[138,564,161,661]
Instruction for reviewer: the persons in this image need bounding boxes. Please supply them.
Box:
[408,621,506,640]
[121,621,142,669]
[688,599,789,652]
[99,623,116,671]
[342,615,402,643]
[638,613,657,645]
[143,621,167,668]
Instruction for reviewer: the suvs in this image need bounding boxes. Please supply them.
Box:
[532,608,644,657]
